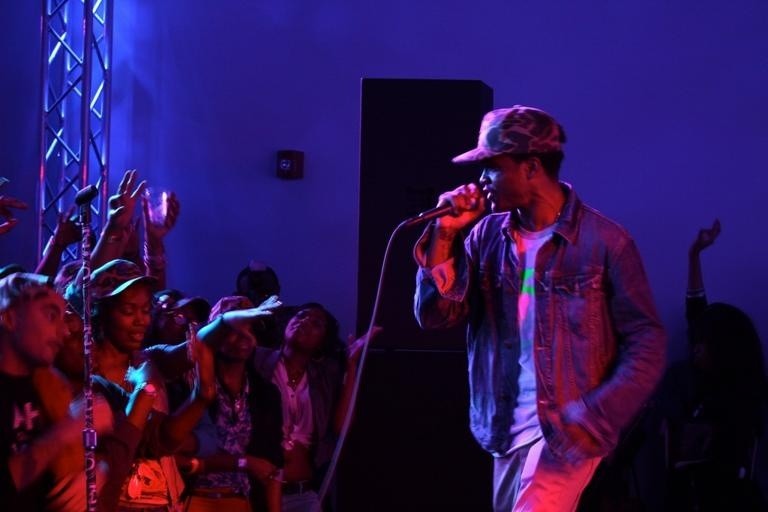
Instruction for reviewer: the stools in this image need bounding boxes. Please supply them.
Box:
[281,480,317,495]
[190,489,245,499]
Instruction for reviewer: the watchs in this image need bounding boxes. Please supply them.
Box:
[135,382,158,398]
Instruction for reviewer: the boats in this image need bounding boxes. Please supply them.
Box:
[355,79,494,350]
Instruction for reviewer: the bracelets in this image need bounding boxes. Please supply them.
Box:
[191,457,200,473]
[267,468,281,480]
[193,376,217,401]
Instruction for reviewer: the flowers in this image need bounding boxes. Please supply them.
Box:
[408,204,453,223]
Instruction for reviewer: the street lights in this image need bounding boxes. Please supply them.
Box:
[118,457,169,510]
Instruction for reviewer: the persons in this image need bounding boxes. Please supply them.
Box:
[411,100,670,511]
[577,212,765,512]
[0,164,387,511]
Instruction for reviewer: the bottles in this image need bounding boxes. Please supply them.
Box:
[88,257,164,302]
[206,294,257,325]
[449,104,563,165]
[150,287,210,315]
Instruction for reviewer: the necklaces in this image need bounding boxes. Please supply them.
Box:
[289,369,303,384]
